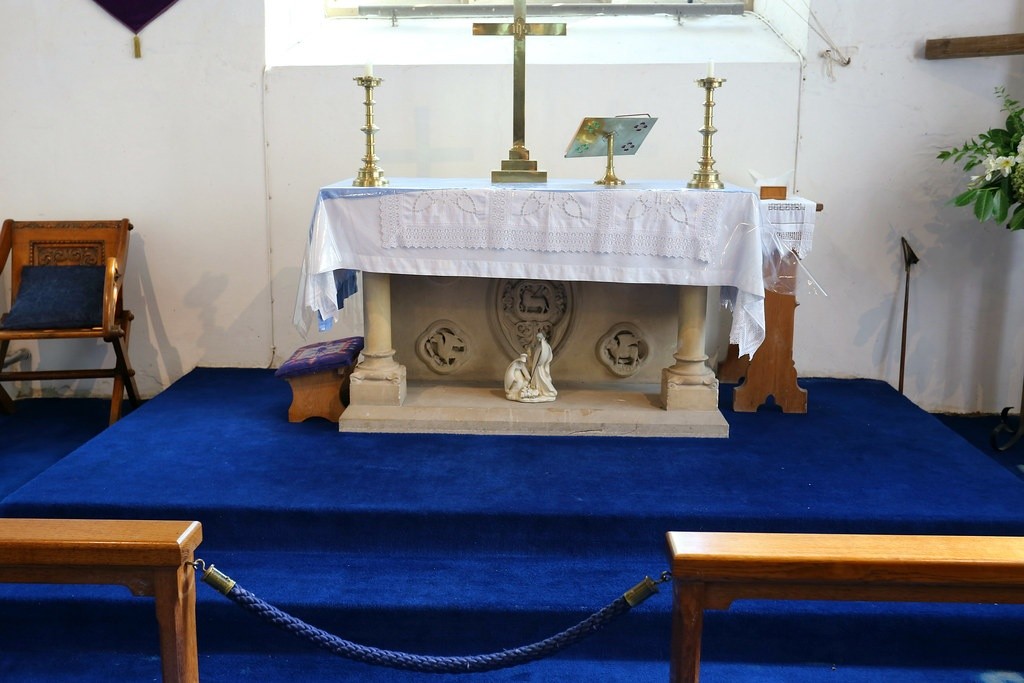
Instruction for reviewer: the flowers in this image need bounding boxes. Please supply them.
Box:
[936,86,1024,232]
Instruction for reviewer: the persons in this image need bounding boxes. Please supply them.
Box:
[531,333,558,398]
[504,353,531,398]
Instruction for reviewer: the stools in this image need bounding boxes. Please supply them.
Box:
[273,336,364,422]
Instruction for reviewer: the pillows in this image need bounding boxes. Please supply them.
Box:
[0,265,106,331]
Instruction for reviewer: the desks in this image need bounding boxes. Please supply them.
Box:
[293,173,823,437]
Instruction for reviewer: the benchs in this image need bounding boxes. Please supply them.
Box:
[0,518,203,683]
[665,531,1024,683]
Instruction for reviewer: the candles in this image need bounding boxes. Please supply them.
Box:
[707,58,714,78]
[364,63,372,75]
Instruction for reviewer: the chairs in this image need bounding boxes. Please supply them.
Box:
[0,218,140,425]
